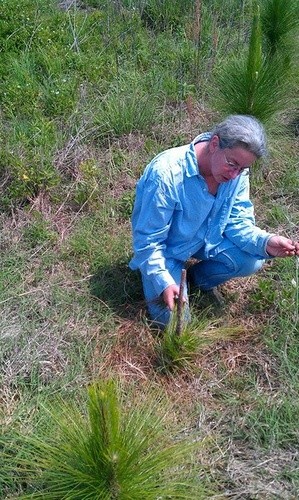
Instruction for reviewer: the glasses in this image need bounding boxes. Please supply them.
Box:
[222,151,250,176]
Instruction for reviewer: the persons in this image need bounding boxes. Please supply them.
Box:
[128,115,299,336]
